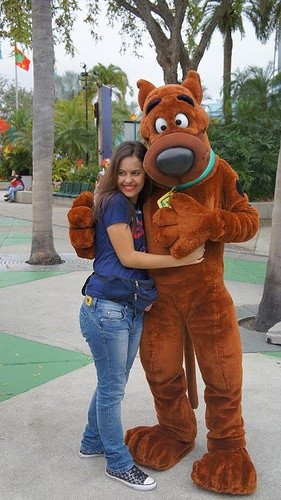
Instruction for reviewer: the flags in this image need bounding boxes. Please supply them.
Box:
[15,48,31,71]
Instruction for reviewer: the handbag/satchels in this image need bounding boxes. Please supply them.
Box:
[94,269,156,312]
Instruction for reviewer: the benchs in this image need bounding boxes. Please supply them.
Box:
[52,182,92,199]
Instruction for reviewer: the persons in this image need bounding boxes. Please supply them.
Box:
[4,170,24,202]
[4,176,24,203]
[78,141,207,491]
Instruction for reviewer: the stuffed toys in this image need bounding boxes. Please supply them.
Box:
[67,66,260,497]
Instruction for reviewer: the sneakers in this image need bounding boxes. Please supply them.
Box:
[76,446,107,458]
[105,461,157,491]
